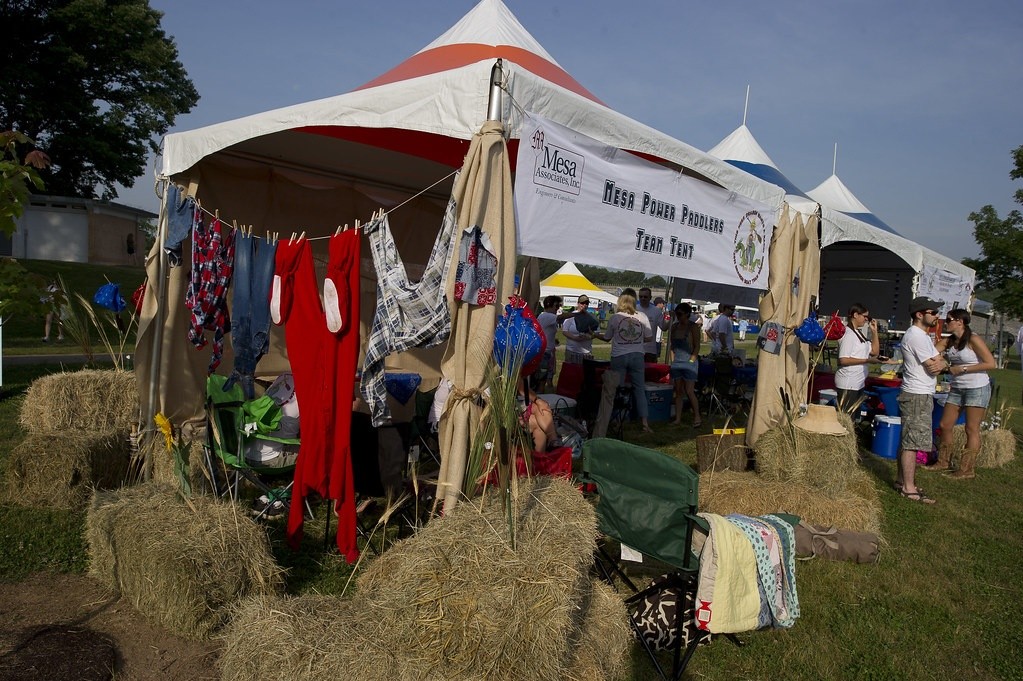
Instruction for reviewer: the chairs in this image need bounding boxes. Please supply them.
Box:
[694,366,750,426]
[204,374,421,559]
[569,437,801,681]
[579,357,631,439]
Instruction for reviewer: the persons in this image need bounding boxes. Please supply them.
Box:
[653,297,667,358]
[593,287,654,434]
[518,377,559,454]
[738,317,750,341]
[562,295,601,364]
[635,288,669,363]
[834,303,879,425]
[536,296,581,393]
[668,303,704,429]
[42,278,64,342]
[882,309,997,480]
[686,300,736,355]
[892,296,945,504]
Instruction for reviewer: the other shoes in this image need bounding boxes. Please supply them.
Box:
[546,439,564,453]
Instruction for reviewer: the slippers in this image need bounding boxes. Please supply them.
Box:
[693,420,703,429]
[666,422,681,427]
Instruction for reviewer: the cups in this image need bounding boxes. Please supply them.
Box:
[941,381,949,392]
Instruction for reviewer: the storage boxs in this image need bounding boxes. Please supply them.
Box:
[643,382,674,421]
[535,393,578,422]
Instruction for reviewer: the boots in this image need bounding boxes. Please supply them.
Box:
[928,442,953,470]
[947,448,978,478]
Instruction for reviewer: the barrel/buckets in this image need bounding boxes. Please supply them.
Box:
[872,414,902,458]
[818,388,837,407]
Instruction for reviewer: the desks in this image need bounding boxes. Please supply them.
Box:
[556,361,670,404]
[698,363,758,387]
[808,370,903,415]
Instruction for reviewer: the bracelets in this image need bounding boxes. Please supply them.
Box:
[963,366,967,374]
[533,396,541,404]
[897,359,903,364]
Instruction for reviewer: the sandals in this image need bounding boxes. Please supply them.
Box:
[641,426,657,434]
[892,480,903,492]
[901,490,935,505]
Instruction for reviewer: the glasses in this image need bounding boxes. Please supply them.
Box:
[554,304,562,308]
[923,310,938,315]
[639,295,649,298]
[946,318,959,323]
[860,313,872,322]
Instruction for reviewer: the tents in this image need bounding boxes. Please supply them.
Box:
[706,122,975,319]
[539,262,619,306]
[135,1,788,522]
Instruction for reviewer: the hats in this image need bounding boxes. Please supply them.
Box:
[910,297,945,314]
[578,294,590,303]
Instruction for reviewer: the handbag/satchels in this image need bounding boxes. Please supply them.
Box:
[552,398,589,459]
